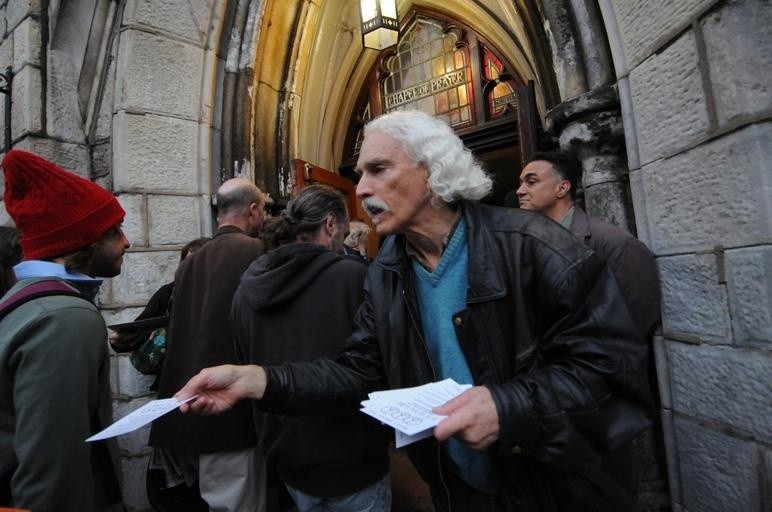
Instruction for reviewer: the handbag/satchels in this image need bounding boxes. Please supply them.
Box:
[130,326,167,375]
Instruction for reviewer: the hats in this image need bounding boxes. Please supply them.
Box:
[1,150,126,261]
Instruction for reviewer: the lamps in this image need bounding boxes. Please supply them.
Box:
[358,1,400,51]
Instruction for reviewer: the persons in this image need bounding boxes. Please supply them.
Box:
[2,150,131,512]
[112,109,660,511]
